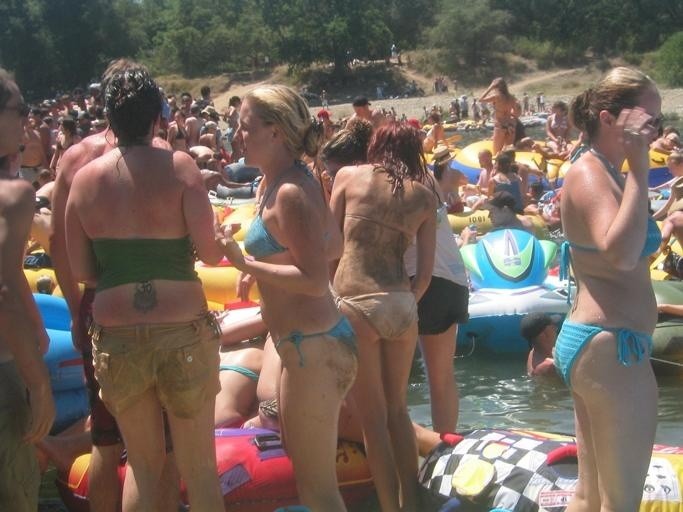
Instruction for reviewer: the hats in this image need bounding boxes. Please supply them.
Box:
[518,313,563,338]
[352,95,371,106]
[317,109,332,119]
[430,145,456,167]
[201,105,216,116]
